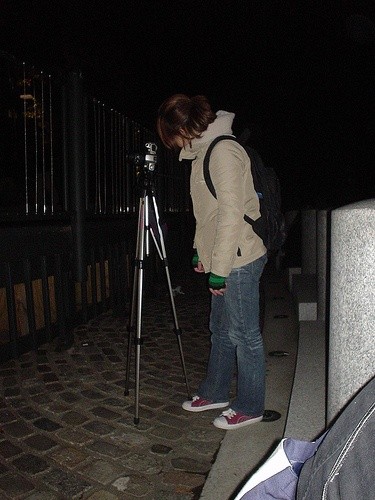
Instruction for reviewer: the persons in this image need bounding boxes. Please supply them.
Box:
[156,94,286,431]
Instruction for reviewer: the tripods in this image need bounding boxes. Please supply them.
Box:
[124,175,194,426]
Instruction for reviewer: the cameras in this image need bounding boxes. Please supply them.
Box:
[143,142,158,171]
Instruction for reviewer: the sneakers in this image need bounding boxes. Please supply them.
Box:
[183,396,228,411]
[213,408,262,430]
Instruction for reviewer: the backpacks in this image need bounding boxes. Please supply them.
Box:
[204,134,285,253]
